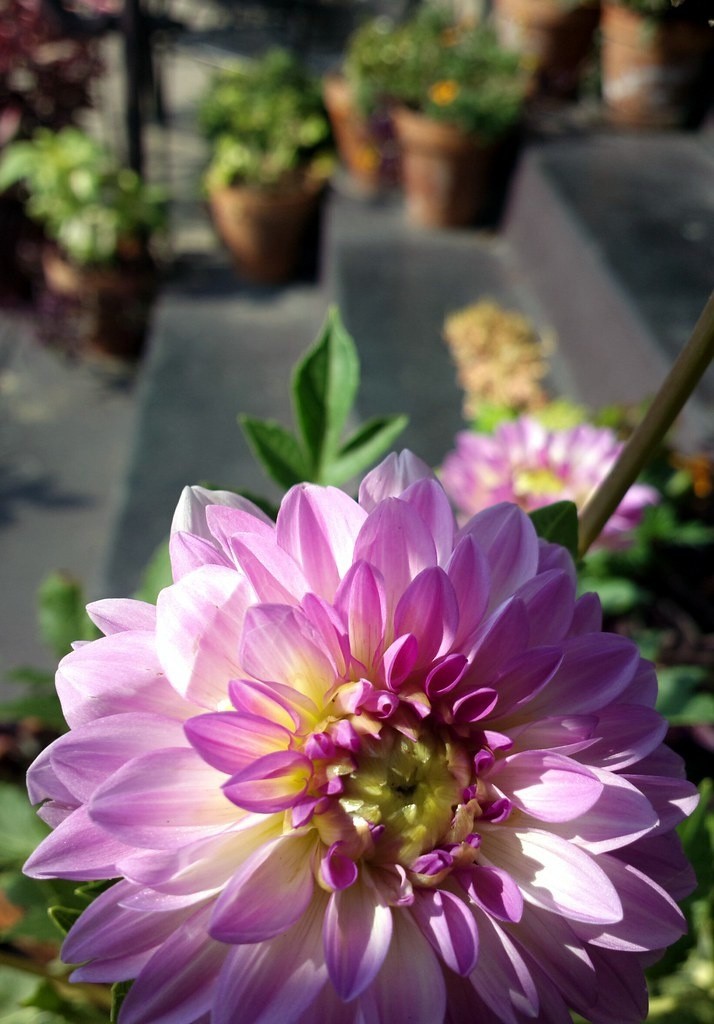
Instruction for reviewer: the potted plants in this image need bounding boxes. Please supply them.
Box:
[320,23,413,182]
[0,127,169,359]
[393,13,529,226]
[600,0,714,132]
[199,46,327,283]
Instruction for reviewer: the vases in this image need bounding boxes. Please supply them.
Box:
[495,0,589,105]
